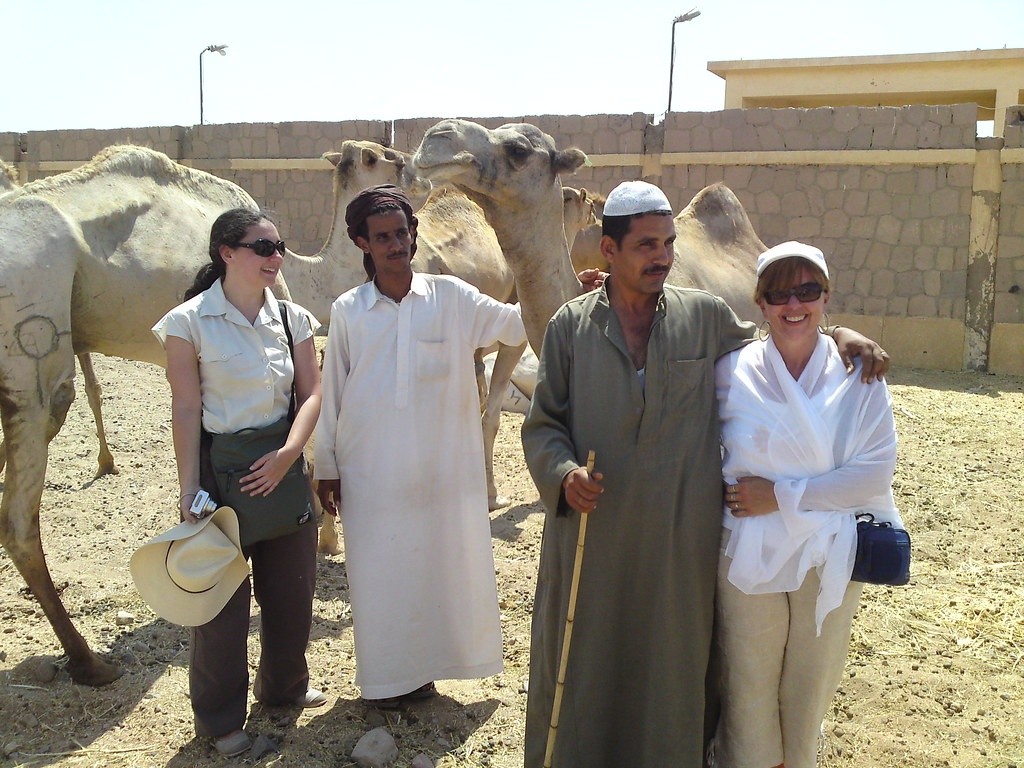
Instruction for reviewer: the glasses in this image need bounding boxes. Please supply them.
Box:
[231,238,286,257]
[762,282,827,306]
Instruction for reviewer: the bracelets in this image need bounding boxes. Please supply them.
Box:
[176,492,197,509]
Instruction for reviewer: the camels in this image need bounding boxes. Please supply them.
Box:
[0,120,770,686]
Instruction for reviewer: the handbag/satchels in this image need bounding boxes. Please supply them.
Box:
[851,512,911,585]
[209,417,315,547]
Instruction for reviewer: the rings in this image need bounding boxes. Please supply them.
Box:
[731,494,736,501]
[881,351,886,354]
[735,502,740,510]
[733,486,735,492]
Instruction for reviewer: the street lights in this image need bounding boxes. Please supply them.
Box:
[669,9,702,115]
[199,43,228,130]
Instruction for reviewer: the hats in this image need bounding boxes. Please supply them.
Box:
[603,181,674,216]
[756,241,829,291]
[129,506,251,626]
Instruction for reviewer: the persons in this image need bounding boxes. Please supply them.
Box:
[712,241,896,768]
[150,209,328,758]
[521,181,890,768]
[312,184,612,708]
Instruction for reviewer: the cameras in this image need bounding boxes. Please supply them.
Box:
[188,488,217,519]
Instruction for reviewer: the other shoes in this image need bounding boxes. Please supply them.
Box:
[214,729,252,758]
[366,694,403,708]
[294,687,327,708]
[410,679,437,699]
